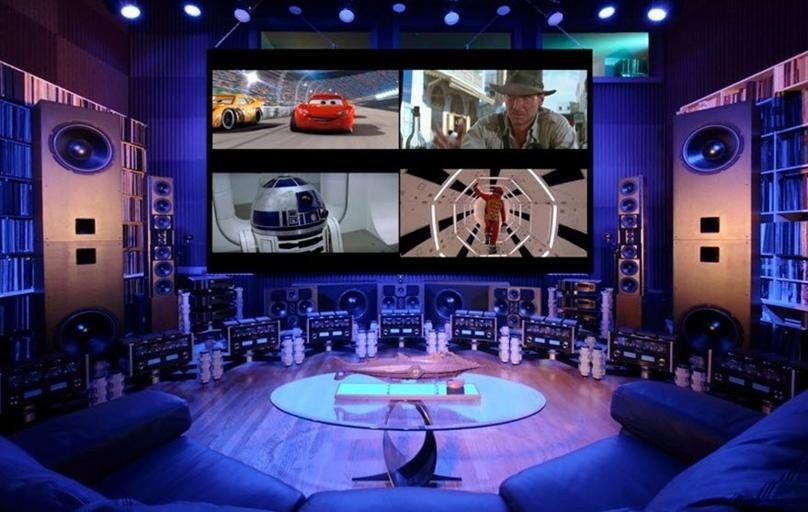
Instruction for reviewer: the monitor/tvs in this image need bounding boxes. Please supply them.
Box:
[207,49,593,276]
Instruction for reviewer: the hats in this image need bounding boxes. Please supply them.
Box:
[488,69,558,97]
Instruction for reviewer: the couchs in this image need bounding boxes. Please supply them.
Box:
[0,377,808,512]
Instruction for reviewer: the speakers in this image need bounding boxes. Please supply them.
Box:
[264,286,318,330]
[377,283,425,319]
[31,99,124,362]
[488,286,541,330]
[614,174,647,330]
[425,282,509,331]
[294,282,377,331]
[670,99,760,352]
[143,175,178,332]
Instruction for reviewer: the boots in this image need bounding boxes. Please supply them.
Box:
[484,236,497,254]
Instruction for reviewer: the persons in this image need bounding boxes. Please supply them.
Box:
[473,183,507,254]
[428,70,580,150]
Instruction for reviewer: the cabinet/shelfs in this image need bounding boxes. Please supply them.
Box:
[0,59,149,373]
[681,45,806,332]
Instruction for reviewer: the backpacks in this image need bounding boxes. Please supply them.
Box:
[485,198,502,223]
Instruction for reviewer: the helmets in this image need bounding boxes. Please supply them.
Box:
[494,187,503,195]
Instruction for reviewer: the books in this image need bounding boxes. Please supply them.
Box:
[755,57,808,105]
[750,86,808,329]
[681,76,774,114]
